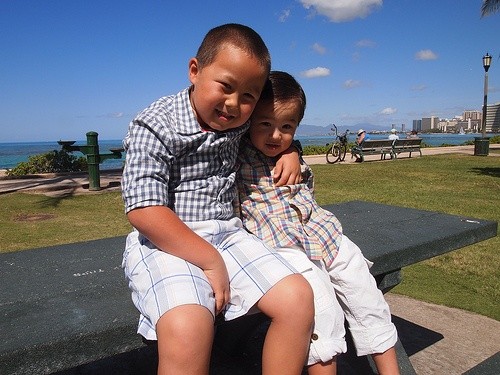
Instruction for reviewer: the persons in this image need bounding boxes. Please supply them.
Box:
[231,71,401,375]
[410,130,419,138]
[120,23,314,375]
[351,129,370,162]
[388,129,399,140]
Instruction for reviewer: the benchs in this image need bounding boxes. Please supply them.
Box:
[384,136,427,160]
[0,198,494,375]
[351,139,395,164]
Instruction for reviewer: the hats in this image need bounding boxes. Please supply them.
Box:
[357,129,366,137]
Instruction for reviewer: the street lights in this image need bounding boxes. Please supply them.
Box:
[480,52,492,138]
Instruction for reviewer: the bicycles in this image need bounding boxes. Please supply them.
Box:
[325,123,349,164]
